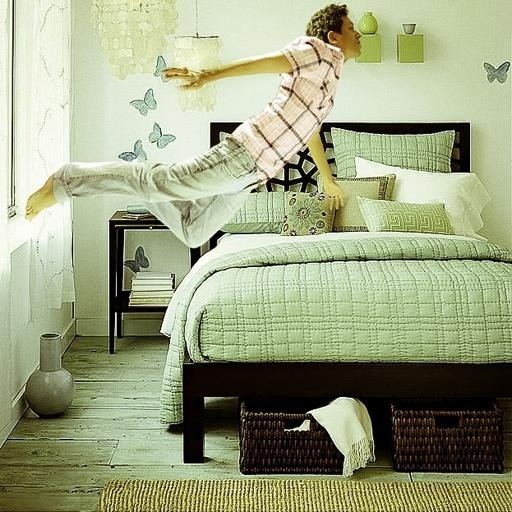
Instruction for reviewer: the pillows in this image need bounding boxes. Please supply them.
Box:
[217,127,491,237]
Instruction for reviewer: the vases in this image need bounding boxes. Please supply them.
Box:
[357,11,378,35]
[26,332,75,418]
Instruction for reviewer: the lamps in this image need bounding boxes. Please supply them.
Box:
[172,0,219,112]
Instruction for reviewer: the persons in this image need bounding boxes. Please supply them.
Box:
[23,1,363,248]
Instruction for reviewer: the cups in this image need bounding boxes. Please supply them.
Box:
[400,22,417,34]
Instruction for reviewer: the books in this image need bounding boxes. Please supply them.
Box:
[126,269,176,309]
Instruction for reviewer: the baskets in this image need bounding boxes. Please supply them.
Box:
[389,399,504,474]
[237,399,344,475]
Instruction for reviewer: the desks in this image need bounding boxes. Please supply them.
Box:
[108,210,201,354]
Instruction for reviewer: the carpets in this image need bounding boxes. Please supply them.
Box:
[96,478,511,512]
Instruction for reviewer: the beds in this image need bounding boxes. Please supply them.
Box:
[181,120,512,465]
